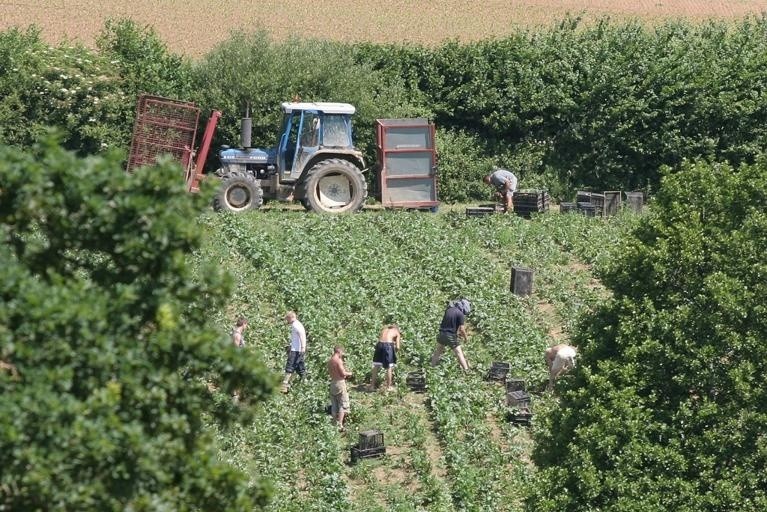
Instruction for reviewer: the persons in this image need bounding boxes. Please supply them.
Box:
[229,317,248,402]
[368,322,401,392]
[545,344,577,388]
[483,168,518,211]
[431,299,472,370]
[280,311,307,391]
[327,344,354,432]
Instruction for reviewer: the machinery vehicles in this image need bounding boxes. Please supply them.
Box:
[207,95,369,215]
